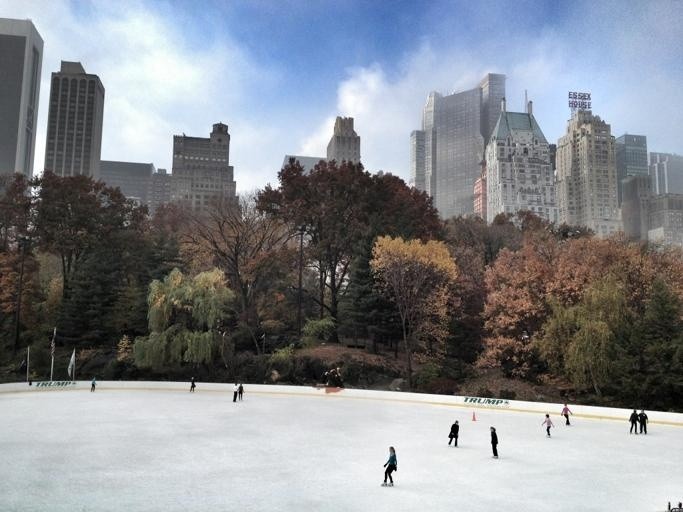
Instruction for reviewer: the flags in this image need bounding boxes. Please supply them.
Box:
[18,345,30,369]
[67,347,75,378]
[47,326,56,360]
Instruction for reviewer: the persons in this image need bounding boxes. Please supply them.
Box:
[561,403,572,425]
[90,377,97,393]
[232,382,239,402]
[189,376,195,393]
[628,408,640,435]
[540,414,555,439]
[489,426,498,459]
[638,407,649,435]
[447,420,458,448]
[382,445,397,485]
[238,382,243,402]
